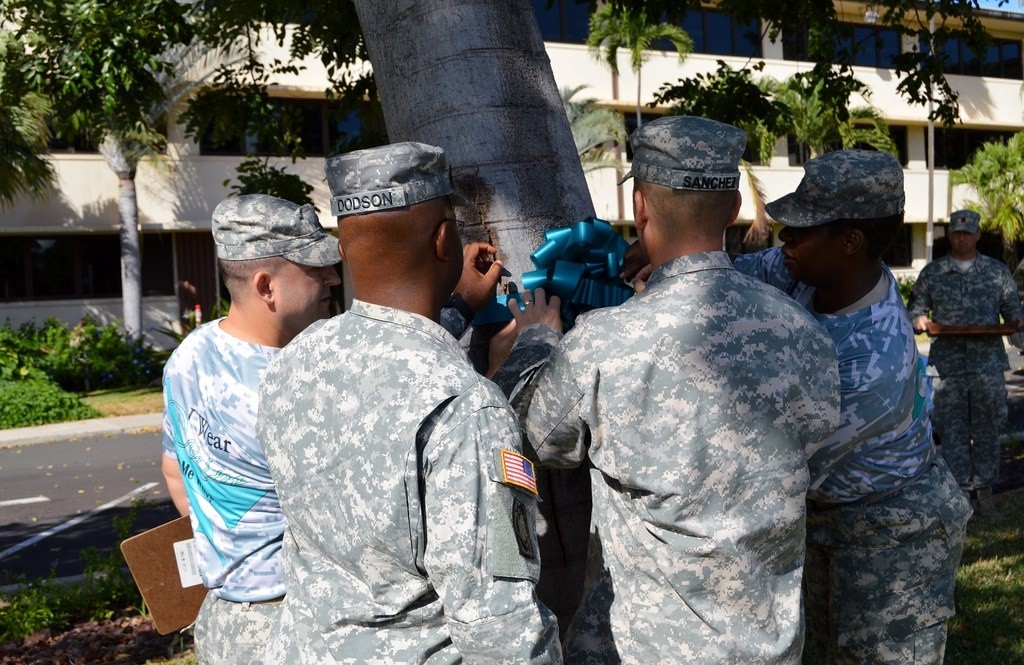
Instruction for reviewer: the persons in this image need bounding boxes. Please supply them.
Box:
[162,143,561,665]
[489,115,975,665]
[906,209,1024,502]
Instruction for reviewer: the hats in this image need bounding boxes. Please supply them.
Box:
[325,141,454,217]
[616,116,749,191]
[765,150,906,227]
[949,210,981,235]
[211,194,344,267]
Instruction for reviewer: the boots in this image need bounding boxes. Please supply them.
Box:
[963,490,971,501]
[975,487,1003,524]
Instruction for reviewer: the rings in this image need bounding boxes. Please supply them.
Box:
[523,300,534,307]
